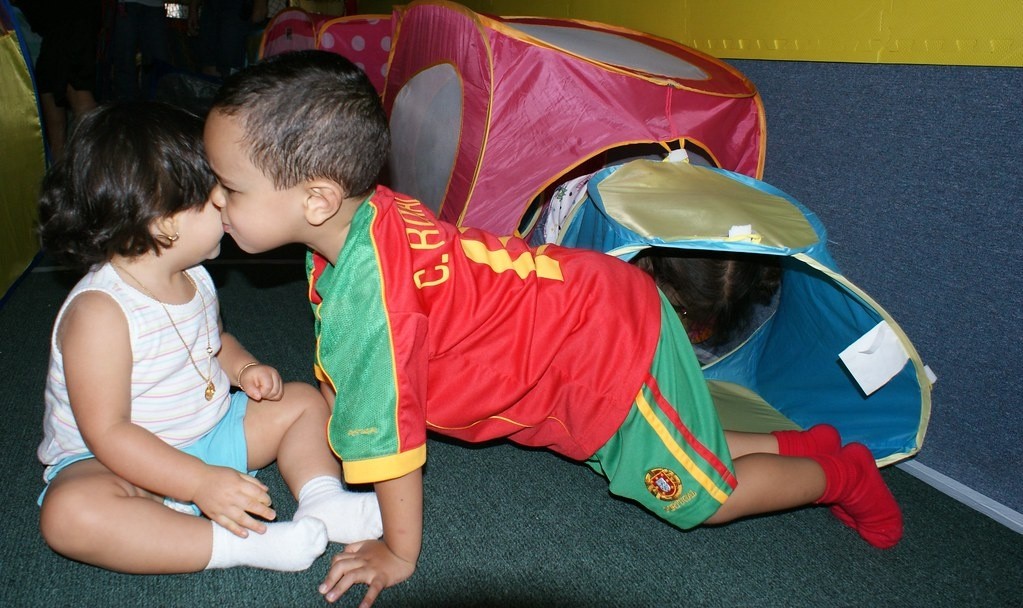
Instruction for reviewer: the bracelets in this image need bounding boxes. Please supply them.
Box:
[237,362,258,391]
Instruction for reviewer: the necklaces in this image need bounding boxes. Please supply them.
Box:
[111,259,216,401]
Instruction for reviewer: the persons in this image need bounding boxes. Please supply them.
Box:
[529,142,786,359]
[36,94,384,575]
[10,0,307,290]
[202,48,903,608]
